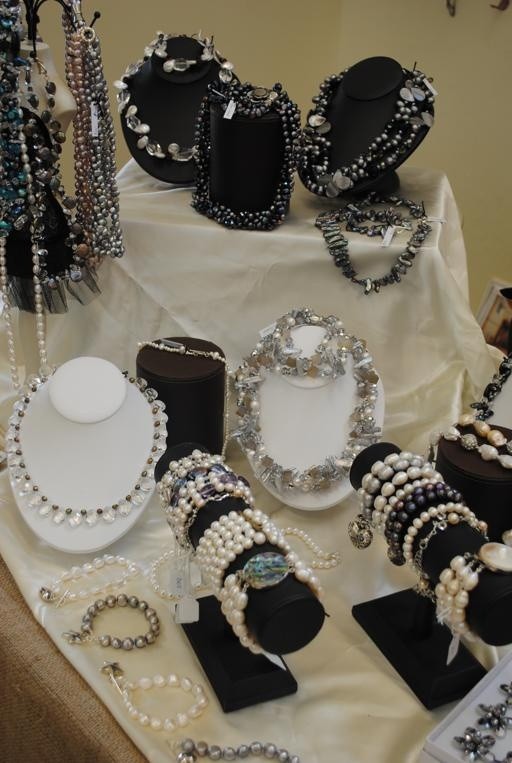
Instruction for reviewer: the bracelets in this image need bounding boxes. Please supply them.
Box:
[157,449,329,656]
[149,548,191,602]
[99,660,207,731]
[347,448,511,644]
[283,524,343,569]
[39,554,140,604]
[174,739,300,763]
[61,596,159,650]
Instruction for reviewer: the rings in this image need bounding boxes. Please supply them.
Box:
[455,680,511,762]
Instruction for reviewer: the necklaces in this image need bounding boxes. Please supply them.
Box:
[295,61,437,196]
[112,27,232,164]
[431,413,511,470]
[138,339,232,456]
[12,367,170,529]
[311,190,433,293]
[190,80,302,231]
[231,305,383,495]
[469,349,512,421]
[1,1,125,389]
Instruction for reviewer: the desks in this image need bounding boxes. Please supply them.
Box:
[1,339,510,763]
